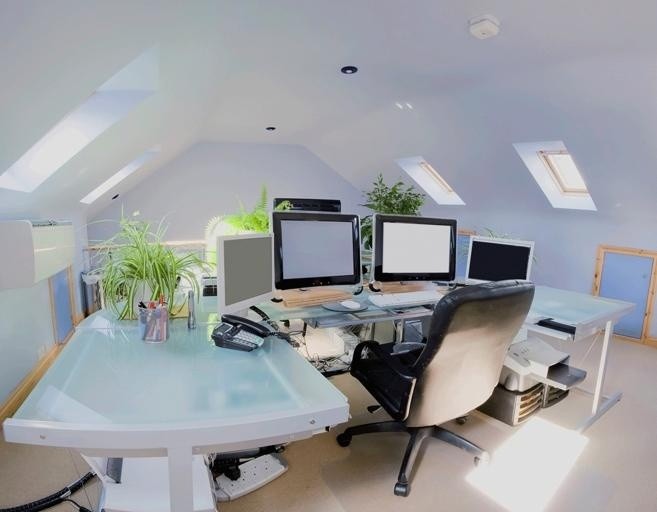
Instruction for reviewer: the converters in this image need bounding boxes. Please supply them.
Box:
[223,462,241,481]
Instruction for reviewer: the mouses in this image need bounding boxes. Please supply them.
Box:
[342,299,360,310]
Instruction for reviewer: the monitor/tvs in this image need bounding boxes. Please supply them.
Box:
[270,210,364,303]
[369,216,459,292]
[272,197,341,212]
[465,235,536,285]
[217,233,274,317]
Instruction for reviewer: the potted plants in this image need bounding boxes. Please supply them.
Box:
[77,199,220,324]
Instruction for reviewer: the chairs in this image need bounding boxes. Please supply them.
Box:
[325,277,536,499]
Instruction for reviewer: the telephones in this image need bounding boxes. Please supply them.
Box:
[211,315,270,352]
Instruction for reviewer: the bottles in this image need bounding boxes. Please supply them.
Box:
[186,290,196,329]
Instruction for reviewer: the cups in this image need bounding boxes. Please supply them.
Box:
[137,301,169,343]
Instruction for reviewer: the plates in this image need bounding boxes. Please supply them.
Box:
[321,301,368,312]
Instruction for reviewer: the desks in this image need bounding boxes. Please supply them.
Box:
[0,270,637,512]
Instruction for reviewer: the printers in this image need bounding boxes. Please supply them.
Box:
[499,338,587,393]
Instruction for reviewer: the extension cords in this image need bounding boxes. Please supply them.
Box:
[216,452,289,502]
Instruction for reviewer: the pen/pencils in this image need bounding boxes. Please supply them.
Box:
[139,294,167,341]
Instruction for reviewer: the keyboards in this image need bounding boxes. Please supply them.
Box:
[368,289,448,309]
